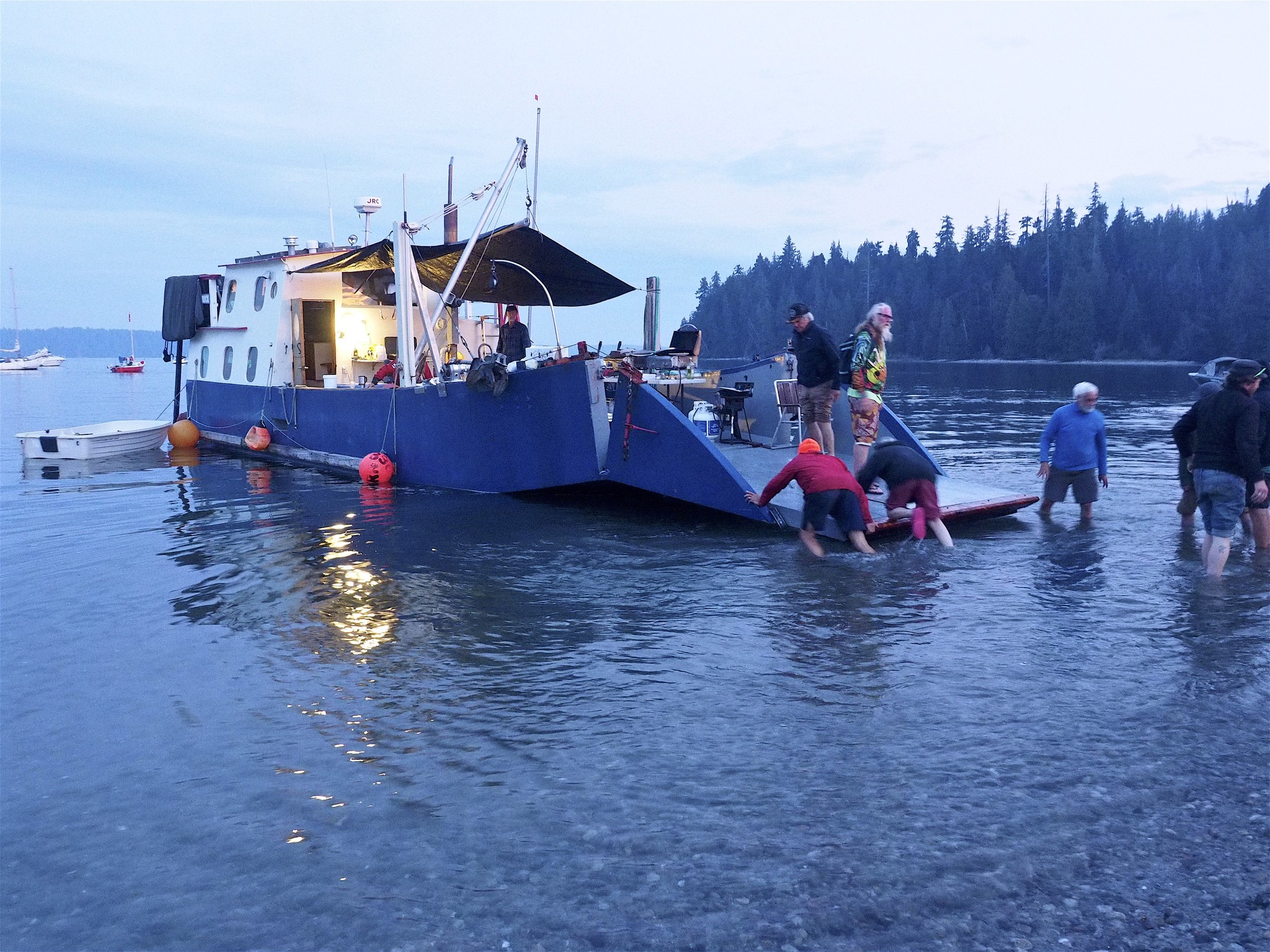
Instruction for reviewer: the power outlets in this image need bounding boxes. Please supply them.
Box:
[342,367,347,374]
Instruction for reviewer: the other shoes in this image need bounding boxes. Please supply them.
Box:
[911,506,926,539]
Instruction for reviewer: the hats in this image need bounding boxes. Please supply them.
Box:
[1231,359,1268,378]
[798,438,821,454]
[1072,381,1097,399]
[506,304,517,312]
[786,302,810,323]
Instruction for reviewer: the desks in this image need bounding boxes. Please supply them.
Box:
[351,358,433,371]
[604,378,707,414]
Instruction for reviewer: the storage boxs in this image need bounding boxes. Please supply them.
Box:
[384,337,417,360]
[525,345,569,361]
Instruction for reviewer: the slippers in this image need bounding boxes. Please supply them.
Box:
[866,484,884,495]
[873,482,880,486]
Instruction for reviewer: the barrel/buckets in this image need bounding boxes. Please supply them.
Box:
[323,374,338,389]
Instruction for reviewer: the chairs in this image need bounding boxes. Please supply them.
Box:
[771,379,834,455]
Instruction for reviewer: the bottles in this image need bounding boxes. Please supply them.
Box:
[661,373,665,380]
[686,357,695,379]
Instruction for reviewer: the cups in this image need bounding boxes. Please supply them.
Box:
[350,381,355,389]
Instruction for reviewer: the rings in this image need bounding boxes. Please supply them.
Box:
[748,497,751,499]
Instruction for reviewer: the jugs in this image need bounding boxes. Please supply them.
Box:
[358,376,367,385]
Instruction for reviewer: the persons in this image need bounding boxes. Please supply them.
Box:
[1172,359,1270,577]
[1036,381,1108,517]
[847,302,893,494]
[855,436,954,546]
[496,306,531,365]
[784,303,841,456]
[744,438,878,557]
[365,337,433,388]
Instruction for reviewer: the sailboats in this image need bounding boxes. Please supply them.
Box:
[110,312,145,373]
[0,267,48,371]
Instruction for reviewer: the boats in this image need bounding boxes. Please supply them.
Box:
[170,102,1041,545]
[35,347,66,367]
[15,420,173,460]
[1187,357,1249,401]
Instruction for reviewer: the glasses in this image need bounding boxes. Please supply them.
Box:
[877,313,894,321]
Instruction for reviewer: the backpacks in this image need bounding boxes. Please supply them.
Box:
[838,328,876,384]
